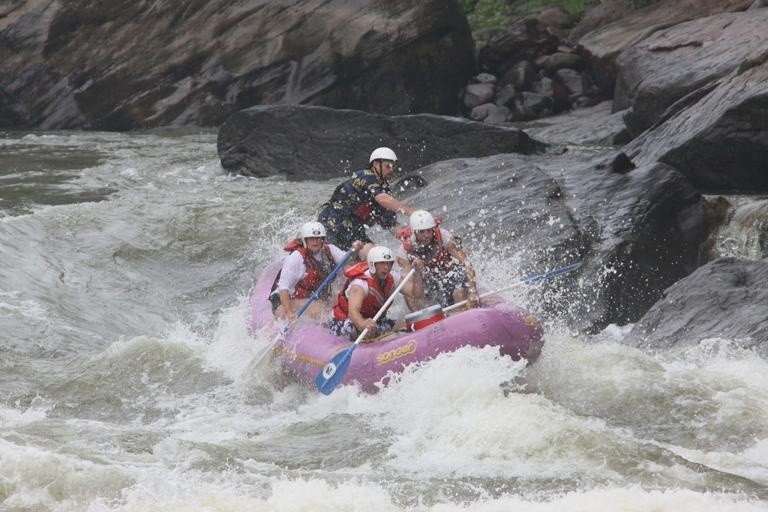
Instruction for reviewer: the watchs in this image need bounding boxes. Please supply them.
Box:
[468,288,477,294]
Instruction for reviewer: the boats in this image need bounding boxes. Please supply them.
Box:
[245,244,544,397]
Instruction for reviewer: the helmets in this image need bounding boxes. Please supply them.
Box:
[409,209,438,244]
[367,245,395,275]
[300,221,327,250]
[368,146,398,164]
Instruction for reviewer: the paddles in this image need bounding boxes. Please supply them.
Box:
[315,266,420,395]
[444,261,583,312]
[240,248,355,375]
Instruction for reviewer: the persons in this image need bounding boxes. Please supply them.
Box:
[317,147,415,262]
[327,245,425,341]
[396,210,480,312]
[269,222,363,322]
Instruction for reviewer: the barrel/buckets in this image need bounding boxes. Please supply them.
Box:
[405,304,445,333]
